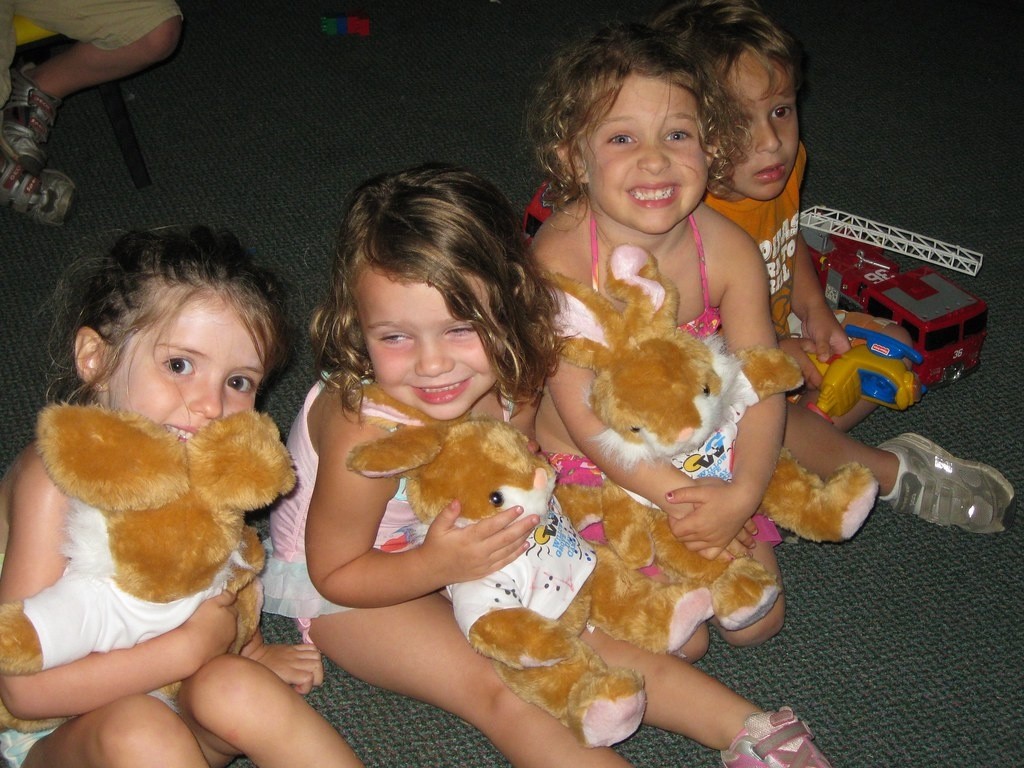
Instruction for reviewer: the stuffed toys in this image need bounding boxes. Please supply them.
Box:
[0,403,297,733]
[541,243,879,631]
[344,384,712,747]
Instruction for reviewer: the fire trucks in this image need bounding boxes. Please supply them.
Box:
[798,205,988,390]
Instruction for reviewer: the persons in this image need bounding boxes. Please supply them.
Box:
[259,163,832,768]
[0,0,184,228]
[0,222,363,768]
[526,21,788,661]
[651,0,1018,534]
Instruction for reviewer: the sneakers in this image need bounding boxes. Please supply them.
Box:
[720,706,833,768]
[0,160,79,226]
[0,60,62,172]
[875,433,1016,533]
